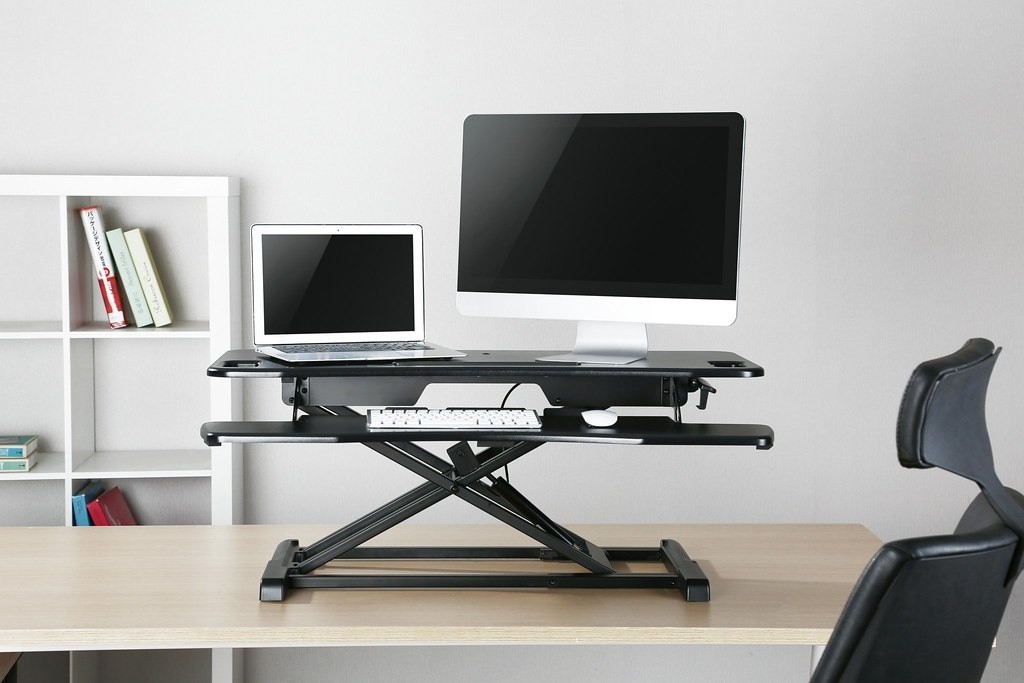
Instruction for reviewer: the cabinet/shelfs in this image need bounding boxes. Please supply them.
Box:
[0,173,245,683]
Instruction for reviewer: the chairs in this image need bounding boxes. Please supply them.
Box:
[810,338,1024,683]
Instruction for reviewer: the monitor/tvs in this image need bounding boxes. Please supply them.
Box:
[456,111,746,365]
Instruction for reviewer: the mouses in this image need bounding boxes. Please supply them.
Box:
[581,409,617,427]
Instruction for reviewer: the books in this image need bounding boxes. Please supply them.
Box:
[0,435,39,472]
[72,479,138,526]
[78,205,173,329]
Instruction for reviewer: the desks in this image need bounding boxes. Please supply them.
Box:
[0,523,996,683]
[199,349,775,601]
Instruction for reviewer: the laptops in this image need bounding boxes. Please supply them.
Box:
[250,223,468,362]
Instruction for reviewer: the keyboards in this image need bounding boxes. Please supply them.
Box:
[366,407,542,429]
